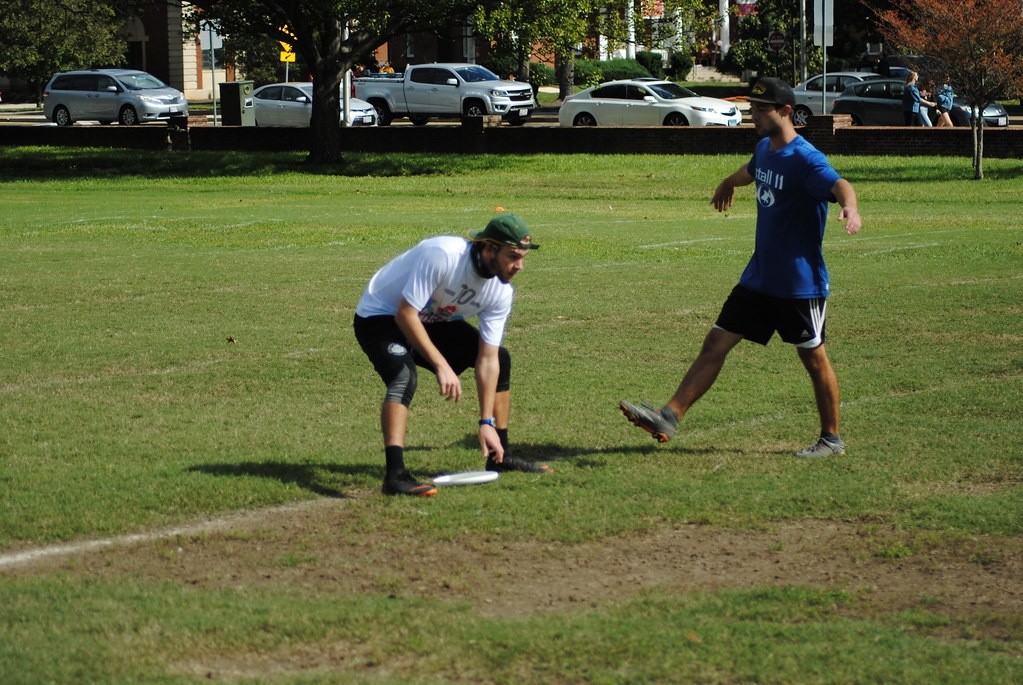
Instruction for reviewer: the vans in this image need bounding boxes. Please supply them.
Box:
[43,68,189,125]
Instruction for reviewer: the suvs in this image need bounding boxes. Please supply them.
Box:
[831,80,1009,127]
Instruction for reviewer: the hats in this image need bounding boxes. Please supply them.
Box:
[746,77,796,108]
[469,214,540,249]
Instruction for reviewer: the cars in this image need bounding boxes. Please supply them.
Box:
[558,78,742,126]
[854,53,911,80]
[253,82,378,128]
[792,72,888,126]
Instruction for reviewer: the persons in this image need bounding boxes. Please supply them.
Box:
[351,62,370,98]
[936,71,959,128]
[377,61,395,74]
[918,77,938,128]
[354,214,552,496]
[902,71,935,127]
[620,76,862,459]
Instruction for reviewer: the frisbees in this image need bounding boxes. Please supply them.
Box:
[432,469,499,486]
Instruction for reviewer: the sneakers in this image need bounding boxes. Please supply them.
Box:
[618,400,676,442]
[381,468,438,496]
[485,449,549,473]
[795,437,845,459]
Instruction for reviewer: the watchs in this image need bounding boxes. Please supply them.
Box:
[478,417,497,427]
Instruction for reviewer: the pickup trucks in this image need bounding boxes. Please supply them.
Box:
[352,62,536,126]
